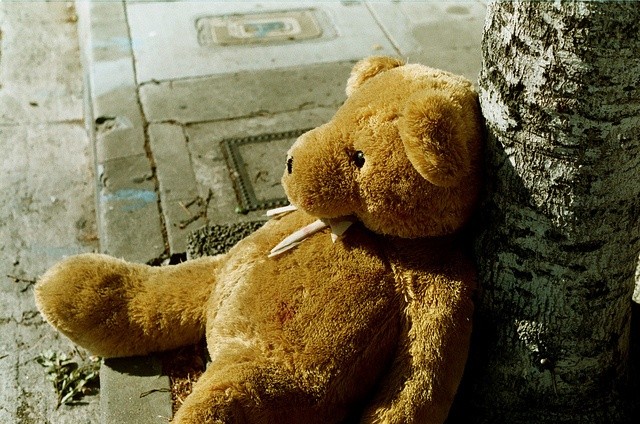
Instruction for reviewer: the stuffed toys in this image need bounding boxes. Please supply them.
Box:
[34,55,479,424]
